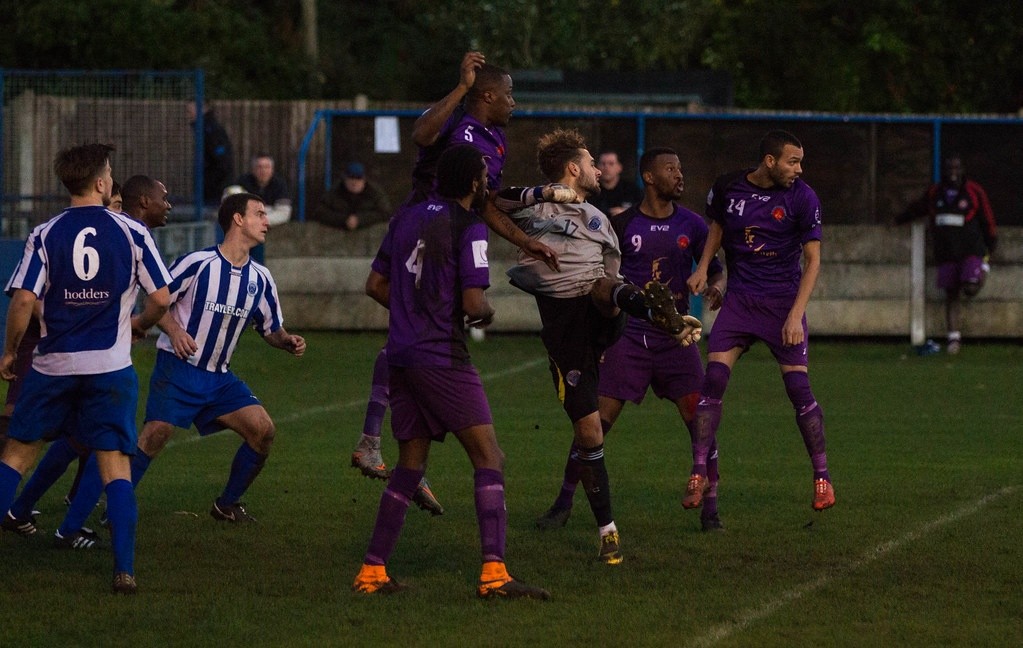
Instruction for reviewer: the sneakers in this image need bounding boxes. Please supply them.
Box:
[352,446,389,480]
[63,495,101,509]
[412,479,446,516]
[5,508,42,537]
[113,571,140,595]
[641,280,692,342]
[811,475,836,512]
[597,531,625,567]
[80,525,98,539]
[535,502,571,532]
[480,575,555,602]
[211,497,259,527]
[698,507,724,532]
[99,517,111,530]
[53,528,98,552]
[680,472,709,511]
[348,575,422,598]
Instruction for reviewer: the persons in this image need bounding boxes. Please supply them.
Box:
[314,161,393,231]
[101,193,308,526]
[681,130,835,510]
[508,129,703,564]
[587,150,640,218]
[352,52,561,514]
[353,147,551,600]
[927,152,999,352]
[239,153,293,226]
[536,147,727,532]
[187,97,237,206]
[0,142,173,593]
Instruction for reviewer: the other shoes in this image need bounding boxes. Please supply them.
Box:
[945,340,961,355]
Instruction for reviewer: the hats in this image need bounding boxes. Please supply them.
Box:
[342,158,369,180]
